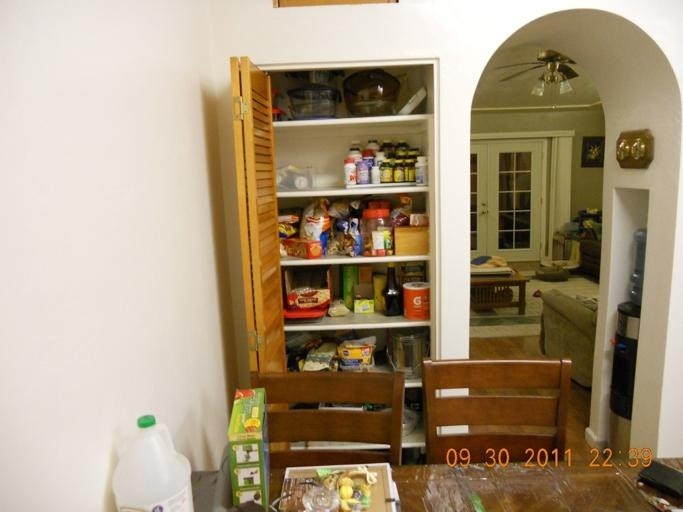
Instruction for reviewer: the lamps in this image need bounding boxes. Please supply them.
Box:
[530,62,572,97]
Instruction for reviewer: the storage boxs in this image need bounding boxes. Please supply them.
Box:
[393,224,428,256]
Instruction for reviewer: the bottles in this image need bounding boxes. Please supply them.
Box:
[342,139,428,187]
[381,262,403,317]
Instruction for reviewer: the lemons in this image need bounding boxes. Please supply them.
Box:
[340,477,354,512]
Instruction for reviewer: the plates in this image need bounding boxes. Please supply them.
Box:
[398,84,427,114]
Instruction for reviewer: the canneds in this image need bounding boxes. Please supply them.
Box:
[403,282,430,321]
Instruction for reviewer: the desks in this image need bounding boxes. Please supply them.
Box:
[470,268,530,316]
[270,461,683,511]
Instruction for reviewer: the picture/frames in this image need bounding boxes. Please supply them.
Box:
[581,135,605,168]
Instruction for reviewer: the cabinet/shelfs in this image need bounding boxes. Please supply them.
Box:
[260,59,438,455]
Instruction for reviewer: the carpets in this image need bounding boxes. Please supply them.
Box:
[470,269,599,324]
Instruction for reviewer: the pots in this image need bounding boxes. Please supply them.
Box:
[286,82,342,121]
[342,68,400,117]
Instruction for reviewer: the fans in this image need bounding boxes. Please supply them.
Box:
[496,50,575,87]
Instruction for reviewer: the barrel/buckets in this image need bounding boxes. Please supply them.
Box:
[110,413,196,510]
[386,327,430,380]
[628,227,647,306]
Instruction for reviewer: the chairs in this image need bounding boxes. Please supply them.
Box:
[422,361,572,464]
[540,288,598,390]
[261,369,405,469]
